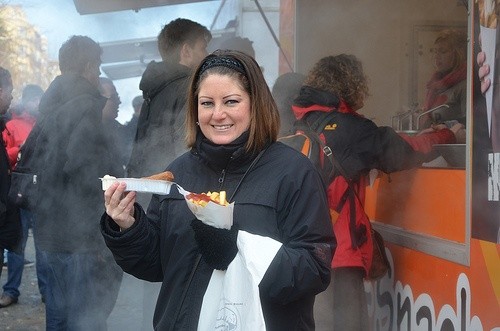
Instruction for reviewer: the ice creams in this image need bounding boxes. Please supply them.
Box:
[478,0,499,138]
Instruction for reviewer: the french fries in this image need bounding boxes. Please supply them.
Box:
[187,190,229,208]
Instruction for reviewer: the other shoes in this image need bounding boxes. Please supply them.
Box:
[24,259,34,267]
[2,262,9,269]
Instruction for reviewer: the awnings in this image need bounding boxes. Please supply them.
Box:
[72,0,223,15]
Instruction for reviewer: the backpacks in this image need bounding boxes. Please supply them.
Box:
[277,110,358,226]
[8,95,103,213]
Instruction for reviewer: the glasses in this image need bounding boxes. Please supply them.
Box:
[104,93,120,101]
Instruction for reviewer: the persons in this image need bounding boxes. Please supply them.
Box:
[271,71,310,140]
[100,50,338,331]
[0,67,24,278]
[0,84,51,307]
[9,35,124,331]
[97,78,133,167]
[417,29,467,144]
[123,95,146,139]
[126,18,213,178]
[276,53,464,331]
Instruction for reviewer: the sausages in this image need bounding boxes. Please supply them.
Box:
[142,171,175,181]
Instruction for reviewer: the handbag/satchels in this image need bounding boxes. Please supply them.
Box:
[368,228,392,282]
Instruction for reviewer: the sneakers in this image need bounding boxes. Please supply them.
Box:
[0,294,14,307]
